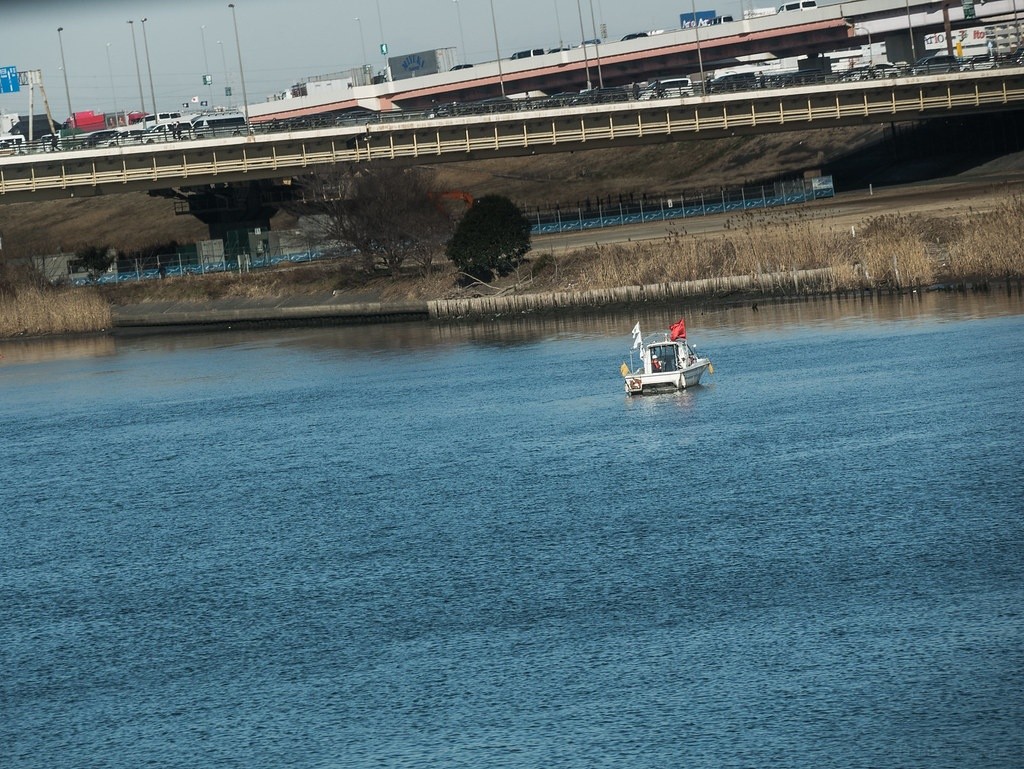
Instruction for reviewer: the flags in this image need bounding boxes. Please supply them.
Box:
[669,319,686,341]
[620,363,629,377]
[632,322,644,361]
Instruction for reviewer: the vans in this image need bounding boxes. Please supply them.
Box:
[142,121,196,144]
[0,135,28,156]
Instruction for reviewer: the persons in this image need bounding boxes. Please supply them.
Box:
[652,355,662,368]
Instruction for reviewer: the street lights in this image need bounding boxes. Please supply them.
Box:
[216,40,231,111]
[141,18,157,123]
[58,26,72,118]
[854,26,873,68]
[201,24,214,114]
[354,17,366,65]
[228,3,250,135]
[106,42,119,127]
[126,20,144,115]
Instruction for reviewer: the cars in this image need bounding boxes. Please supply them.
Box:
[776,0,818,15]
[450,64,473,71]
[0,143,16,156]
[710,72,756,93]
[911,55,959,76]
[960,56,1000,72]
[838,64,902,81]
[620,32,648,41]
[420,85,629,120]
[41,131,118,148]
[108,130,150,147]
[337,111,379,125]
[192,112,247,140]
[706,15,733,26]
[639,78,694,100]
[510,39,601,60]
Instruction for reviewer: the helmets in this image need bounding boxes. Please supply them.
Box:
[652,355,657,359]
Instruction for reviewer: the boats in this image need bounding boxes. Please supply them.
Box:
[624,333,710,396]
